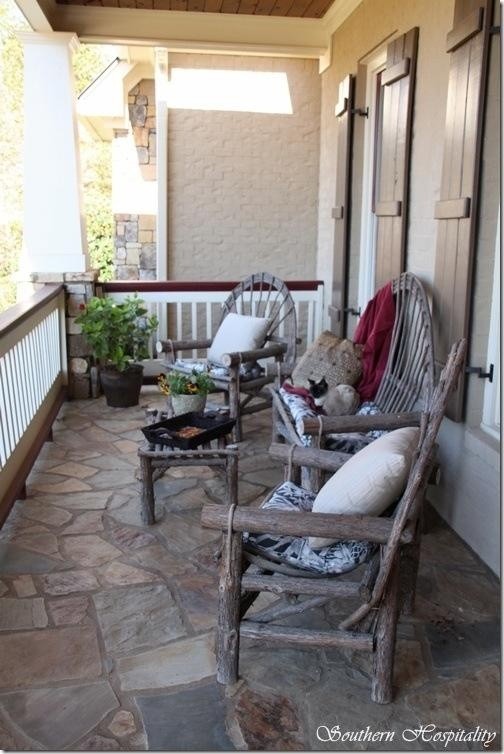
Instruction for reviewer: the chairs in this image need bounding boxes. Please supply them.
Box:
[153,270,299,445]
[198,341,475,708]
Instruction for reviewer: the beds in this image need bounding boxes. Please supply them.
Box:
[136,405,240,528]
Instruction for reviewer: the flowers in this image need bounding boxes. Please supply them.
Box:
[154,364,216,397]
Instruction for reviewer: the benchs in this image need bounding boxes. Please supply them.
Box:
[267,273,434,493]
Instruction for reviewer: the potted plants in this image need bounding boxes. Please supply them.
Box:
[74,291,159,408]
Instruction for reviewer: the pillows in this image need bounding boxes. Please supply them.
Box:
[287,331,367,396]
[240,481,379,575]
[306,427,421,550]
[205,313,275,374]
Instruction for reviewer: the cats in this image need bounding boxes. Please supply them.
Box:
[307,376,361,416]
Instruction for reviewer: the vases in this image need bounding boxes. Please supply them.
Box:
[171,394,207,416]
